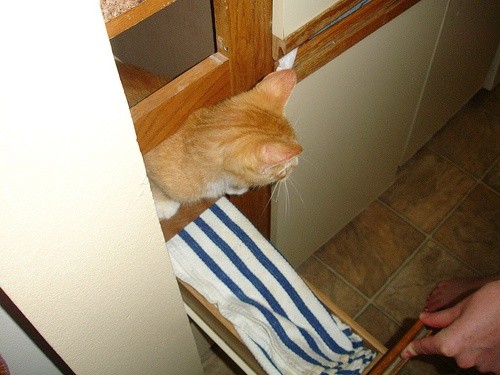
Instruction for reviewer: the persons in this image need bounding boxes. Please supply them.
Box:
[401,277,500,375]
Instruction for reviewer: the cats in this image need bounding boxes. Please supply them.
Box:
[114,60,305,224]
